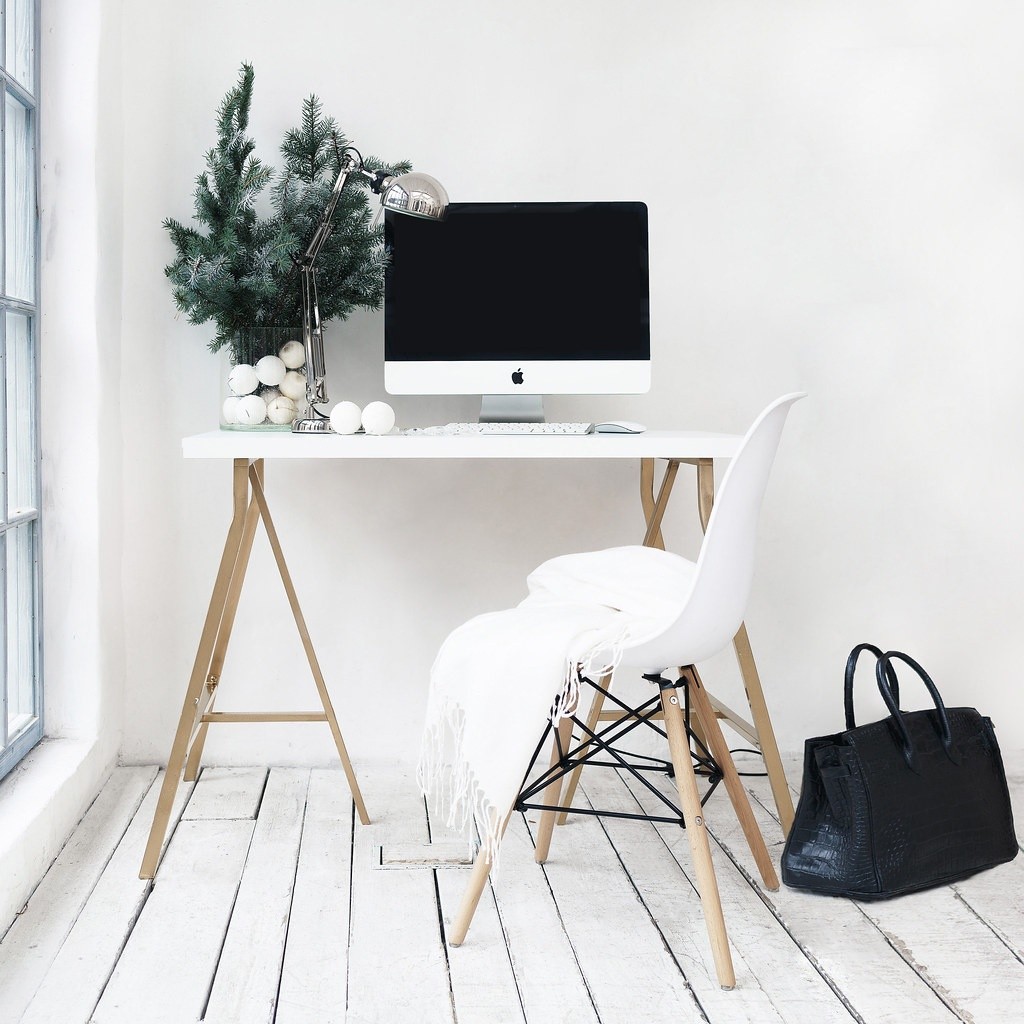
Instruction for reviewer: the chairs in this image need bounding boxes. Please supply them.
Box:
[457,390,808,986]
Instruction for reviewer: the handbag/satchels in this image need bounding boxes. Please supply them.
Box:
[780,644,1018,901]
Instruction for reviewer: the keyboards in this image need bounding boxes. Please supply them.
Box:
[404,421,596,436]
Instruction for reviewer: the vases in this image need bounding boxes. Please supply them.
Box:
[228,324,309,430]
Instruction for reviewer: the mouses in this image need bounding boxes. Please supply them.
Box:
[596,421,645,433]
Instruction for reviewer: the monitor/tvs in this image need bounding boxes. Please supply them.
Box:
[383,201,650,424]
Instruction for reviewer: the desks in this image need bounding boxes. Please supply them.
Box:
[134,422,797,887]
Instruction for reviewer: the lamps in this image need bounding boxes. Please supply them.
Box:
[290,146,451,432]
